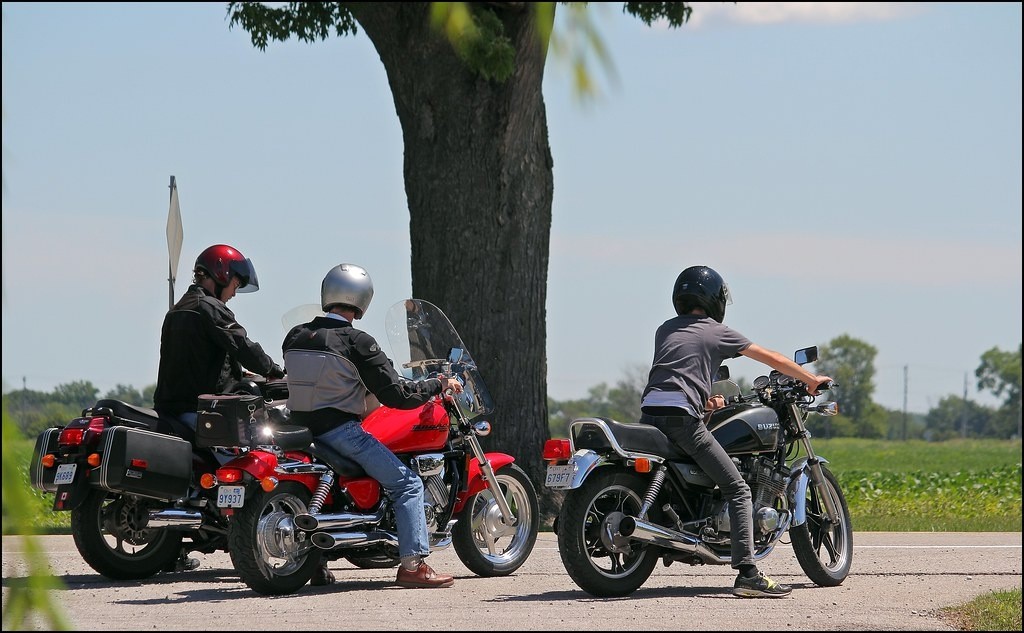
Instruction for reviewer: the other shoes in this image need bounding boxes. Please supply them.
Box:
[161,553,201,572]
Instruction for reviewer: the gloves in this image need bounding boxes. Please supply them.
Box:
[244,362,284,383]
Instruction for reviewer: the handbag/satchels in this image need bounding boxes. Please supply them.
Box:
[196,392,267,445]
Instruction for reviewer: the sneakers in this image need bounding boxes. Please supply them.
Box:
[732,570,792,599]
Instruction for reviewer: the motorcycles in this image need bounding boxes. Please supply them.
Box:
[194,298,541,598]
[28,372,291,583]
[541,345,855,596]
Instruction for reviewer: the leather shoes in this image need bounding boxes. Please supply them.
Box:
[310,568,335,586]
[395,560,454,588]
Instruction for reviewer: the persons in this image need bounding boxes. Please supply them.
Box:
[282,263,463,588]
[153,244,285,572]
[639,266,835,597]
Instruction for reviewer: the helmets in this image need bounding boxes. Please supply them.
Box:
[321,264,373,320]
[672,267,726,323]
[192,244,250,288]
